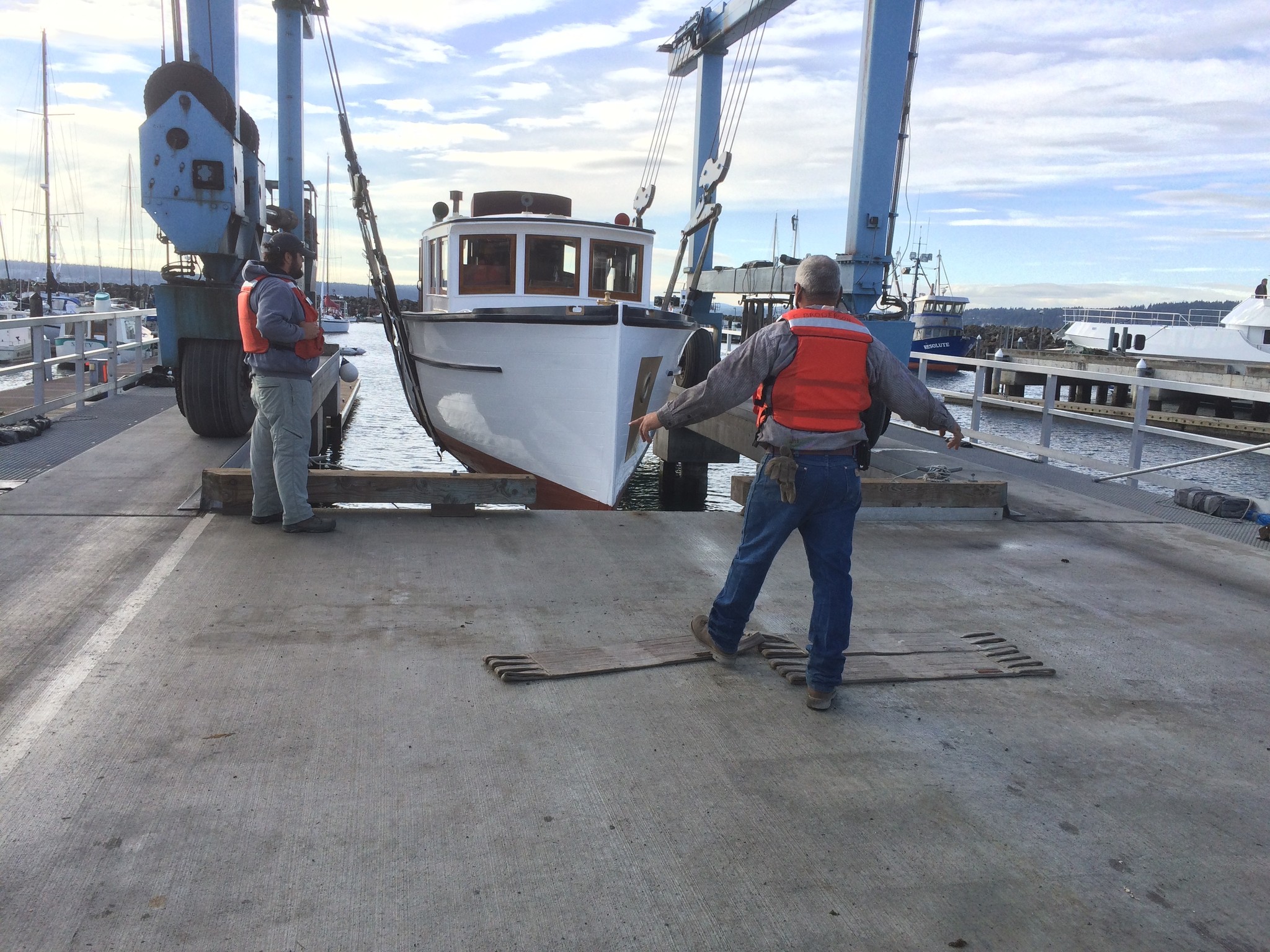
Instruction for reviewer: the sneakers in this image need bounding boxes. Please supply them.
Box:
[807,683,837,710]
[250,510,283,524]
[282,516,336,532]
[690,614,738,666]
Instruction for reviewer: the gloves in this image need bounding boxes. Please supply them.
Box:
[764,444,798,504]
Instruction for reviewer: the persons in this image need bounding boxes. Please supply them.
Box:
[238,232,337,532]
[627,255,965,709]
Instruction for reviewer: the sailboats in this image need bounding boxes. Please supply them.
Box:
[316,155,350,332]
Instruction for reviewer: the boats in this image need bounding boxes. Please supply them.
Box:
[0,29,139,371]
[902,225,978,372]
[1064,294,1270,364]
[398,189,699,510]
[54,292,155,364]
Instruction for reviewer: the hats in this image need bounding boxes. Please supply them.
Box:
[264,232,315,257]
